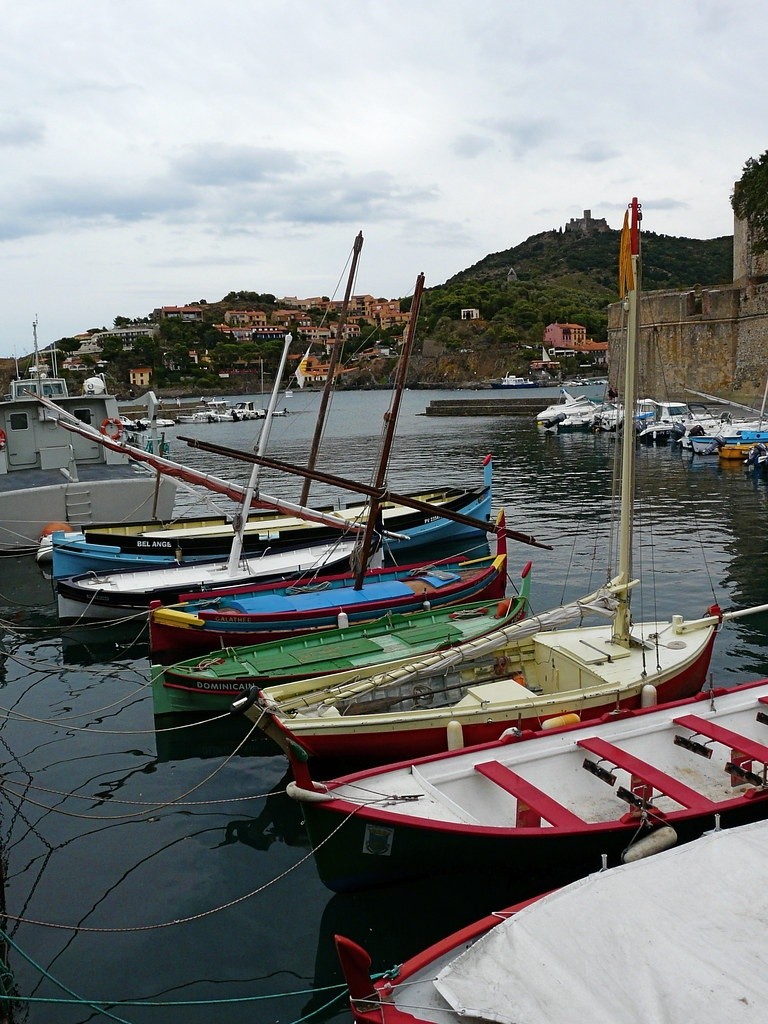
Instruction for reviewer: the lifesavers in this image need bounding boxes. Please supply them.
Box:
[230,686,258,716]
[101,418,123,440]
[0,428,6,449]
[692,283,704,298]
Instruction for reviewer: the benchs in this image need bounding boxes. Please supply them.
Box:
[474,715,767,828]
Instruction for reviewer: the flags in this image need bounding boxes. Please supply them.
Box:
[295,348,310,389]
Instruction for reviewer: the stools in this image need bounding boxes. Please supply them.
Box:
[467,675,539,704]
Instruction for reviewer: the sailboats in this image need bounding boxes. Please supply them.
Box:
[49,454,495,587]
[225,197,768,774]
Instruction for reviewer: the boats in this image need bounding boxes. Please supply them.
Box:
[536,389,768,469]
[163,558,534,715]
[490,372,540,388]
[285,680,768,861]
[537,370,610,387]
[0,312,179,558]
[332,818,768,1024]
[147,505,510,660]
[120,400,290,432]
[55,332,388,627]
